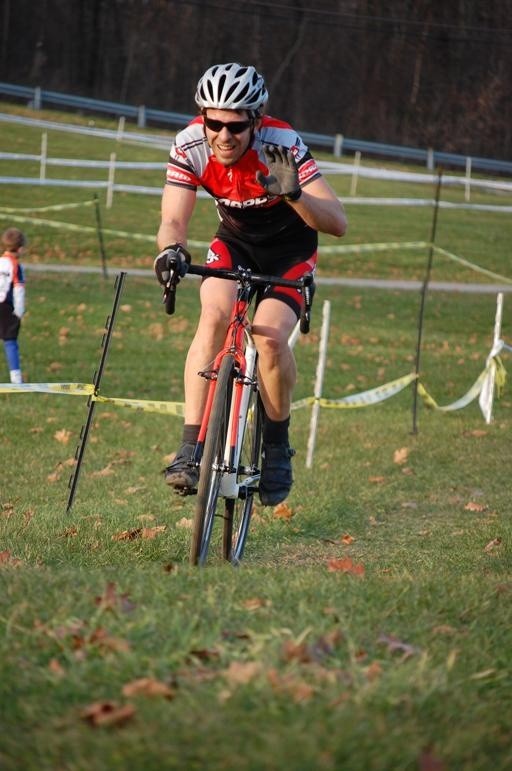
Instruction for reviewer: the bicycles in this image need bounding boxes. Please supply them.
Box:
[158,248,317,573]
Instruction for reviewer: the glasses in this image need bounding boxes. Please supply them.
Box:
[203,116,254,135]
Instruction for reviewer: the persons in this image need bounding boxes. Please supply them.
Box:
[0,224,29,385]
[156,60,350,506]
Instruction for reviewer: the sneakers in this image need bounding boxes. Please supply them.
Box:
[258,439,296,508]
[165,438,205,491]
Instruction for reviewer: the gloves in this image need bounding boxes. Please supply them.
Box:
[151,243,189,289]
[251,143,307,204]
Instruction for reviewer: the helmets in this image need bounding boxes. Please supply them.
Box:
[193,62,270,114]
